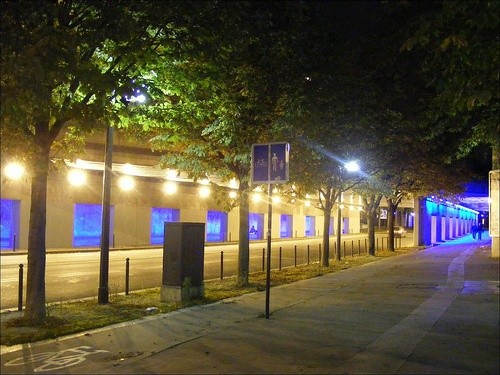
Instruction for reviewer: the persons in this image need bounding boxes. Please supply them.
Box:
[249,226,255,233]
[477,223,483,240]
[471,223,477,240]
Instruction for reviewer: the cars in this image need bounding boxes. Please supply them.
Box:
[387,226,407,238]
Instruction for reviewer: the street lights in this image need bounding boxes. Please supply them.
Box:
[98,87,146,306]
[337,161,358,260]
